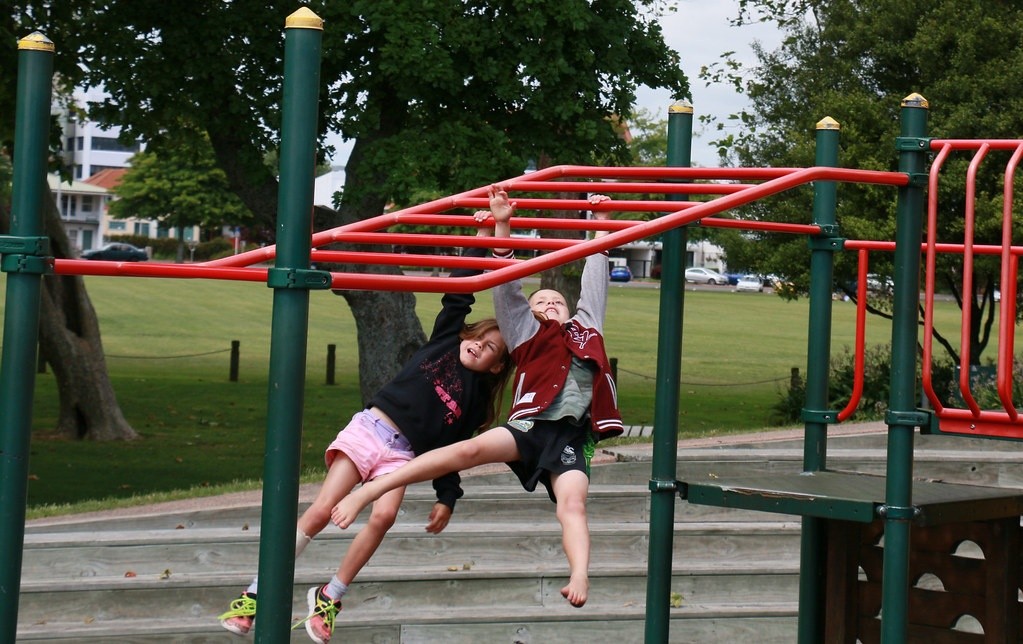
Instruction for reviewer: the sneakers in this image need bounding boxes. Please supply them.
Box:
[217,590,257,636]
[290,582,343,644]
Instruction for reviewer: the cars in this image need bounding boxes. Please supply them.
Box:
[736,274,765,294]
[865,273,895,295]
[80,242,151,264]
[720,269,781,285]
[609,266,632,282]
[685,268,730,286]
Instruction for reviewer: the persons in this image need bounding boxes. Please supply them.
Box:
[331,183,624,608]
[217,210,519,644]
